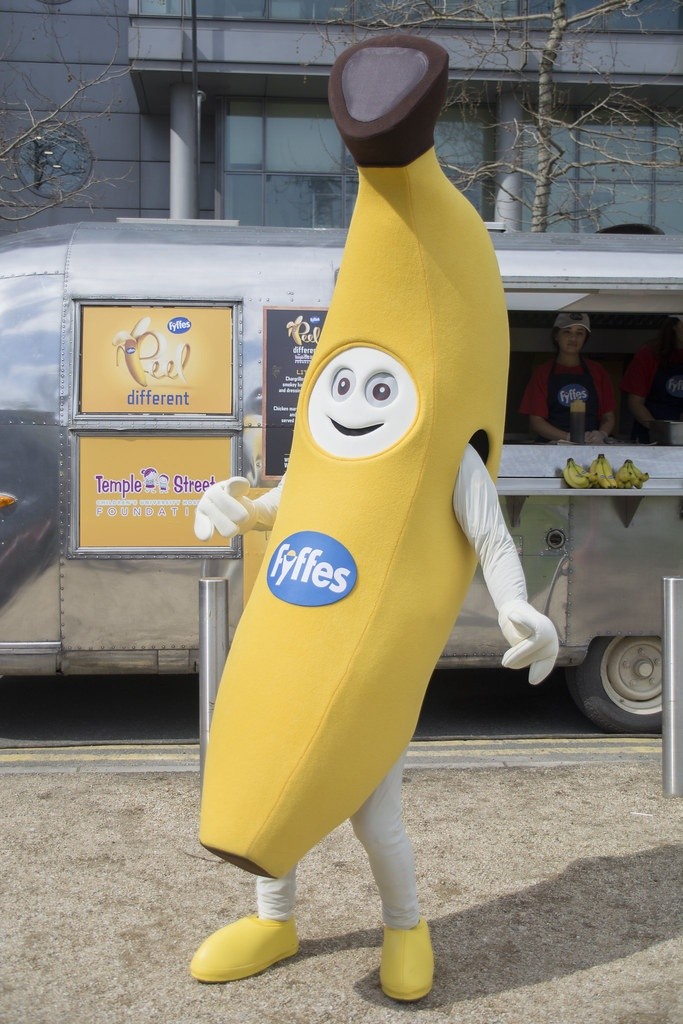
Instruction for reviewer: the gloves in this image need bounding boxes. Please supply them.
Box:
[587,430,607,443]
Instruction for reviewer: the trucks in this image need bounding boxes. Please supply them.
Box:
[0,215,683,734]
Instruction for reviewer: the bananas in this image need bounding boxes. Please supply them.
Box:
[589,454,617,489]
[196,34,506,879]
[614,459,649,490]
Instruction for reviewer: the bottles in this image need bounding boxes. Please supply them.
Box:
[570,397,586,443]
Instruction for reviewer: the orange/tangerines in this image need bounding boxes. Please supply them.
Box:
[563,457,598,488]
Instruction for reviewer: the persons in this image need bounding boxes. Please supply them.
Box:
[618,312,683,444]
[517,312,620,445]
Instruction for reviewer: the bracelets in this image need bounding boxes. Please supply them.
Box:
[566,431,570,440]
[600,430,608,438]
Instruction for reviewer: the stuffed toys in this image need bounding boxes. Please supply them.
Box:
[185,41,562,1002]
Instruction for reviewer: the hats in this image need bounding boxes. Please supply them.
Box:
[554,313,591,333]
[668,314,683,322]
